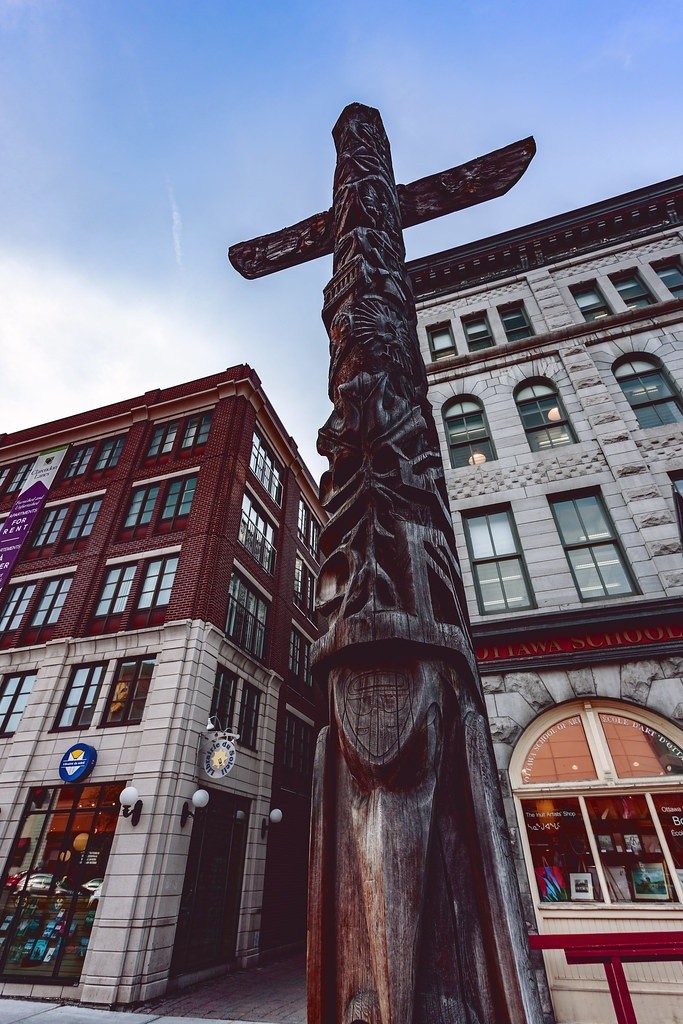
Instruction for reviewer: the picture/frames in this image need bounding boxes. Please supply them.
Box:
[630,862,670,901]
[569,872,595,900]
[587,865,632,901]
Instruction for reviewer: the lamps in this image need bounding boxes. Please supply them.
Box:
[261,808,283,841]
[180,788,209,829]
[467,449,487,466]
[206,715,236,746]
[118,786,143,828]
[547,407,562,421]
[233,810,245,826]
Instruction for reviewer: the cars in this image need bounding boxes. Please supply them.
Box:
[79,878,104,910]
[7,869,67,910]
